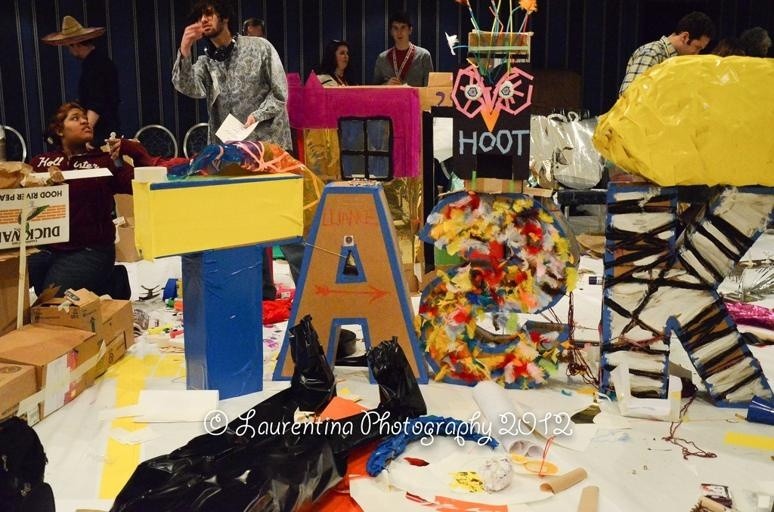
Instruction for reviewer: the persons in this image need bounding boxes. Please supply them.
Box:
[370,11,437,88]
[710,34,747,58]
[613,9,716,104]
[317,39,364,87]
[64,37,123,147]
[168,0,309,303]
[25,99,157,300]
[739,25,774,59]
[241,14,270,41]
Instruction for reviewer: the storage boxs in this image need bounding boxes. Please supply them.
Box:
[1,246,135,431]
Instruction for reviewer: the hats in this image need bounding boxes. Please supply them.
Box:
[40,15,106,48]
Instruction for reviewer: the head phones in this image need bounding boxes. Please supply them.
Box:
[204,31,238,61]
